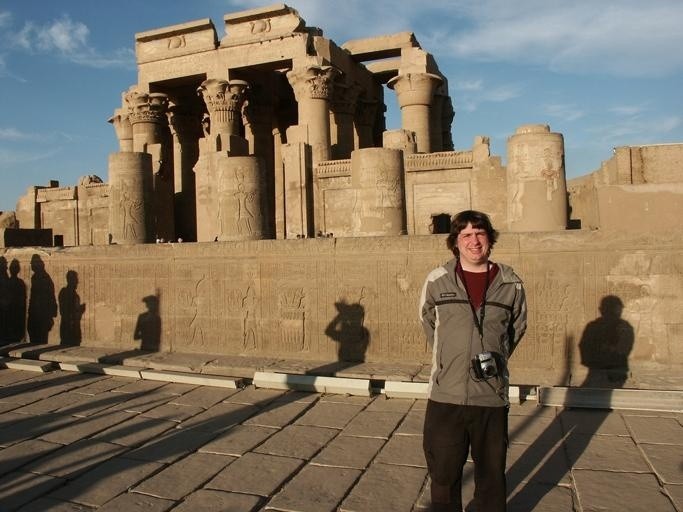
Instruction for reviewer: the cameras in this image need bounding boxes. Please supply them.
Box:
[471,351,502,379]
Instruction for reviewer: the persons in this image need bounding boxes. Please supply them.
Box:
[419,210,527,512]
[297,230,333,239]
[156,234,184,243]
